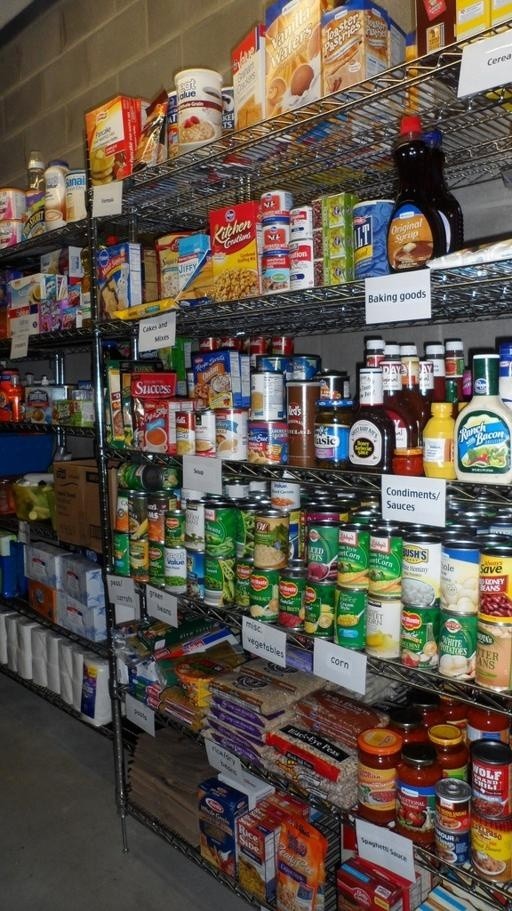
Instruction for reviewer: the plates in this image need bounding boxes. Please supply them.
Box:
[88,19,512,911]
[0,218,124,735]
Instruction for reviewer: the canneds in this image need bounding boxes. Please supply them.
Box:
[260,218,289,251]
[290,240,316,291]
[260,250,290,296]
[175,408,249,459]
[319,374,352,400]
[106,476,511,691]
[259,190,293,219]
[313,399,355,469]
[287,207,313,240]
[358,686,512,884]
[394,447,423,475]
[248,356,285,465]
[285,354,321,380]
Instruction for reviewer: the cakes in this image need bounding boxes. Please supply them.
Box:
[285,354,321,380]
[175,408,249,459]
[259,190,293,219]
[394,447,423,475]
[248,356,285,465]
[260,218,289,251]
[287,207,313,240]
[358,686,512,884]
[290,240,316,291]
[260,250,290,296]
[313,399,355,469]
[319,374,352,400]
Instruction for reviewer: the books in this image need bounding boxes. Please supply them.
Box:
[49,458,119,557]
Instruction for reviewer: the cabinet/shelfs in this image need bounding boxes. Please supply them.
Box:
[7,374,27,422]
[451,353,511,484]
[22,151,45,237]
[43,158,69,233]
[348,337,466,479]
[384,114,447,270]
[424,126,464,255]
